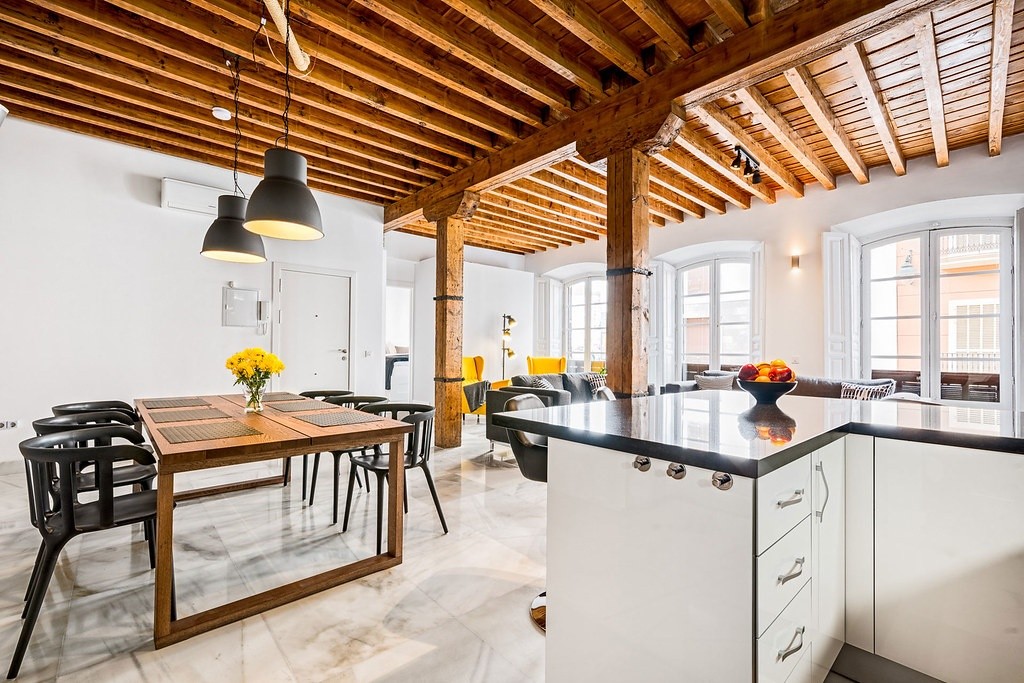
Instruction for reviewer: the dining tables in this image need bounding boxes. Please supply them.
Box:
[132,392,415,651]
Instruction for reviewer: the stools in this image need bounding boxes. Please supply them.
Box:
[503,393,548,632]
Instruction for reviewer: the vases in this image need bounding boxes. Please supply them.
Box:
[242,379,269,413]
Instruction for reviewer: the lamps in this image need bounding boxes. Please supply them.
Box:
[241,0,325,242]
[200,48,268,263]
[730,145,763,185]
[502,313,517,380]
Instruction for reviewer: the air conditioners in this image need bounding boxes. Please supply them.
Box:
[160,177,252,218]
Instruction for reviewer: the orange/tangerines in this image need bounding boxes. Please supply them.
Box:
[754,359,787,382]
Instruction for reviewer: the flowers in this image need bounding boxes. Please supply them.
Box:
[225,347,287,411]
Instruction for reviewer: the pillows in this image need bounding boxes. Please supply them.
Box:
[840,382,897,401]
[386,344,409,354]
[586,373,608,401]
[532,377,555,389]
[693,374,734,391]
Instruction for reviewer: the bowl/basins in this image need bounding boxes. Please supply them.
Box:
[737,379,797,403]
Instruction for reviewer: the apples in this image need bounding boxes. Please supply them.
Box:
[769,367,792,382]
[738,363,759,380]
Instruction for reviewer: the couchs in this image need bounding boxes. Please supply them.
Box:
[659,369,920,402]
[486,372,655,453]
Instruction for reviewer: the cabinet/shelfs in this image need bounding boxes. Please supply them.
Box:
[753,452,813,683]
[810,435,846,683]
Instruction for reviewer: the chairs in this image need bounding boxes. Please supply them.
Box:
[596,386,616,402]
[342,403,448,555]
[284,390,363,501]
[309,395,390,524]
[23,411,158,602]
[461,355,512,425]
[527,356,567,375]
[50,401,143,473]
[5,425,176,680]
